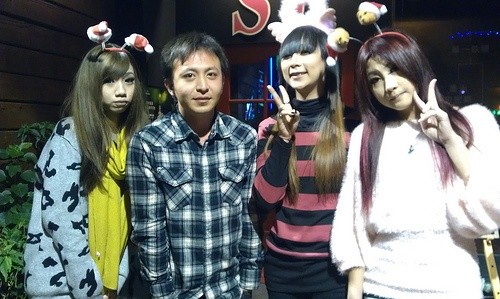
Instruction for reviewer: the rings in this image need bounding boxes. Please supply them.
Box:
[283,101,290,104]
[278,113,283,119]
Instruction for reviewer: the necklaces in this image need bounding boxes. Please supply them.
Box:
[401,118,426,155]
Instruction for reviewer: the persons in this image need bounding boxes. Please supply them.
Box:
[23,20,154,299]
[246,25,366,299]
[126,32,263,299]
[329,31,500,299]
